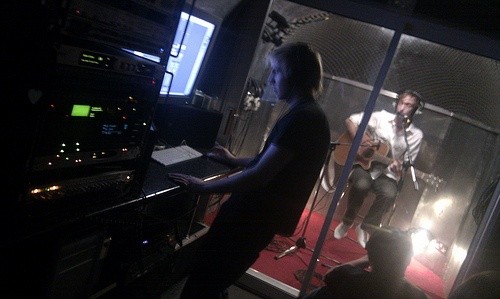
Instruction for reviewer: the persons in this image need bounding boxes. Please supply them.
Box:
[324,227,499,299]
[169,42,331,299]
[333,89,427,248]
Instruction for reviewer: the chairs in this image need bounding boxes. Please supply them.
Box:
[328,165,404,244]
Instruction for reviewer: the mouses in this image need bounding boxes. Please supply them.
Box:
[207,151,219,157]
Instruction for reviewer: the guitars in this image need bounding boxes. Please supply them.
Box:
[332,132,444,192]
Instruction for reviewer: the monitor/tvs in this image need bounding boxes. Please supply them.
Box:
[157,4,223,104]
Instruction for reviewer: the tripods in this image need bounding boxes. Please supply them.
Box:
[274,142,367,265]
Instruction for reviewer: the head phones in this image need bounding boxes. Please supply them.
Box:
[393,90,424,119]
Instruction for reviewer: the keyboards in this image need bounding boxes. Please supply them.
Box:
[150,145,202,166]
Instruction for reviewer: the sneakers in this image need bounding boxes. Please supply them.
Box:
[334,220,353,239]
[354,223,369,248]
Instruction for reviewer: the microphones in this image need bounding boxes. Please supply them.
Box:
[364,143,380,147]
